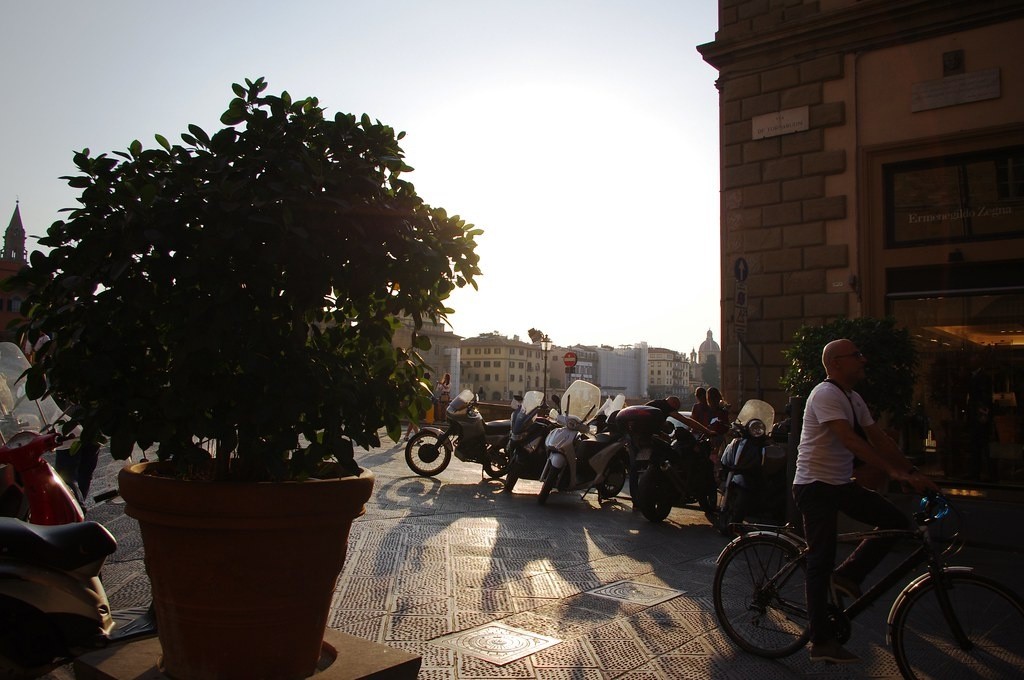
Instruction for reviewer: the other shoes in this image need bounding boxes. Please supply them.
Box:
[810,638,861,663]
[831,572,863,600]
[418,440,423,444]
[404,437,409,440]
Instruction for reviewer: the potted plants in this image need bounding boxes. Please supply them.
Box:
[2,75,484,680]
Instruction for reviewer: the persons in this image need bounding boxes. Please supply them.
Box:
[423,372,436,404]
[403,422,424,444]
[49,409,101,502]
[436,373,450,423]
[792,337,941,662]
[690,388,729,433]
[629,396,716,512]
[20,329,51,383]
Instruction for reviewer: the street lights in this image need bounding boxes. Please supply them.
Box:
[541,334,552,411]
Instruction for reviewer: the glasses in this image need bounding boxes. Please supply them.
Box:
[835,351,864,362]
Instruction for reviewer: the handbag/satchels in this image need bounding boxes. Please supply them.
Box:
[826,379,871,468]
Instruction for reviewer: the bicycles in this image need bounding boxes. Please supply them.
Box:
[713,487,1024,680]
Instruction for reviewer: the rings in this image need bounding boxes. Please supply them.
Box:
[916,478,919,481]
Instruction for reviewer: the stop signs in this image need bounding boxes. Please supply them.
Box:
[563,352,577,367]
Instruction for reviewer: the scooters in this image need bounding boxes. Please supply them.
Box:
[711,399,792,532]
[504,390,558,491]
[614,402,733,521]
[0,342,85,525]
[0,458,160,680]
[539,380,626,500]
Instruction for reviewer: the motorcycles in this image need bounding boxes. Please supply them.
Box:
[404,389,511,478]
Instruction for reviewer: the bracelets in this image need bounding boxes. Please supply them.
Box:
[909,466,920,474]
